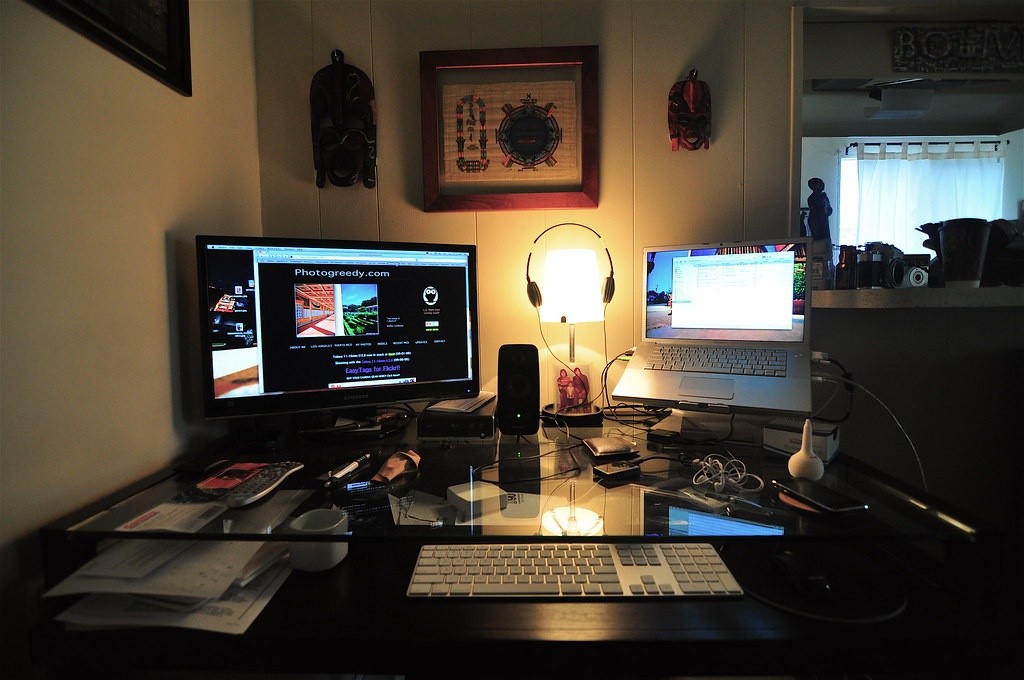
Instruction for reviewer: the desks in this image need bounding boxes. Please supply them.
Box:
[39,412,1024,680]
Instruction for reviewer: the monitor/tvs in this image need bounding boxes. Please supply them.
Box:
[195,234,480,442]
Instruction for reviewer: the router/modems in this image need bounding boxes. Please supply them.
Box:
[417,395,497,442]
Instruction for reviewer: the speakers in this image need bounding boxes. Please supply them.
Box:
[499,434,540,496]
[496,344,539,436]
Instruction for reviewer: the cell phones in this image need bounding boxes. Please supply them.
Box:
[773,477,871,512]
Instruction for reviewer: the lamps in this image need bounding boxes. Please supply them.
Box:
[538,249,603,427]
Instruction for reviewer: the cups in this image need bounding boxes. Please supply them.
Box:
[937,218,992,288]
[288,508,349,572]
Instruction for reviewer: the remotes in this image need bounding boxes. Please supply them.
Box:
[227,460,305,510]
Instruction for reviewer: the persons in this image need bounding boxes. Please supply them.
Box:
[556,368,591,414]
[807,178,835,270]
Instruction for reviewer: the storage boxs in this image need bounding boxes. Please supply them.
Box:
[762,417,841,466]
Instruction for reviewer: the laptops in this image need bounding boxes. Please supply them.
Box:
[631,487,797,535]
[611,237,813,417]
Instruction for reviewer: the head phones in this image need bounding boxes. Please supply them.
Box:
[526,223,614,308]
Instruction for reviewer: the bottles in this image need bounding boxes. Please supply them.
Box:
[835,245,857,290]
[787,418,825,482]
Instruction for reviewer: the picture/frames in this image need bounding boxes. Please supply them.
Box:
[552,361,593,414]
[26,0,193,97]
[420,45,601,212]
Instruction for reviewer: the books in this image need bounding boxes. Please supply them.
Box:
[133,543,290,612]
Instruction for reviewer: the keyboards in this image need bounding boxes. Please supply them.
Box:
[405,543,744,596]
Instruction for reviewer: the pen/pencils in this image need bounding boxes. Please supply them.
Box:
[346,480,379,490]
[324,454,371,488]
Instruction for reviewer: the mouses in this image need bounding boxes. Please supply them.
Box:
[781,550,837,603]
